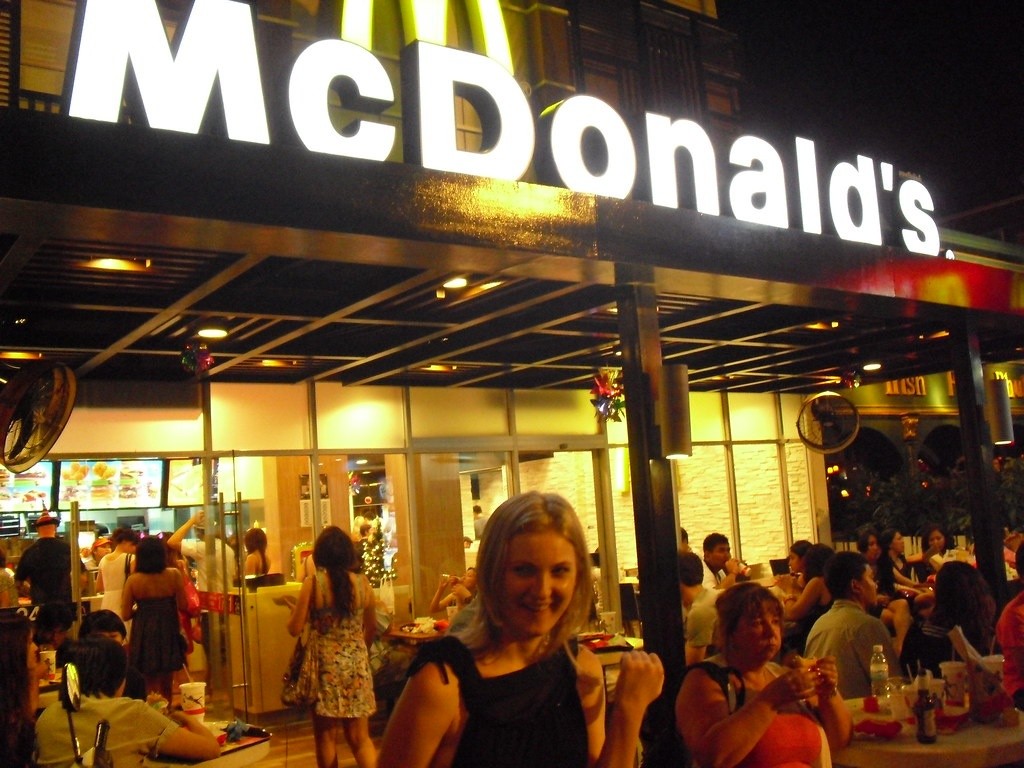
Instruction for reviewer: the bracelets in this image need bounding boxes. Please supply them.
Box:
[785,595,797,604]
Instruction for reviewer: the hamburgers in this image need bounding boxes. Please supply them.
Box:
[793,655,818,668]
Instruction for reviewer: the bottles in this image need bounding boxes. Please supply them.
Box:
[914,668,936,743]
[869,644,890,705]
[599,612,616,634]
[440,574,461,584]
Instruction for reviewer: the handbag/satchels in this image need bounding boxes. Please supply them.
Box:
[638,661,745,768]
[281,630,320,708]
[379,577,394,615]
[179,561,201,617]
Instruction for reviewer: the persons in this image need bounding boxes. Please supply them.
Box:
[363,623,410,719]
[996,542,1024,712]
[679,527,834,649]
[78,610,146,702]
[464,537,473,548]
[0,523,138,667]
[353,511,376,543]
[284,526,377,768]
[157,510,271,668]
[0,611,48,768]
[430,567,477,613]
[899,561,997,684]
[856,523,956,655]
[35,640,220,768]
[803,551,904,700]
[675,582,851,768]
[376,490,664,768]
[473,505,489,540]
[121,535,188,695]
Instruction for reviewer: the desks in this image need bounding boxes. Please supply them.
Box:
[34,667,65,709]
[819,695,1024,768]
[389,630,446,659]
[145,719,272,768]
[581,635,644,711]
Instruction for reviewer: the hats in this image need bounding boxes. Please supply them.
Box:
[193,519,218,529]
[92,537,112,550]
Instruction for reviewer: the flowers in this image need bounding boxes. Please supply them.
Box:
[590,365,627,423]
[182,343,212,376]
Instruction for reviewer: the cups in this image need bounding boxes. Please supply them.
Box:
[902,685,916,728]
[180,681,207,725]
[939,662,967,706]
[39,650,56,681]
[982,656,1006,684]
[447,606,458,622]
[738,563,753,577]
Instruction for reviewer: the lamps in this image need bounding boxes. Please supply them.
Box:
[986,379,1015,445]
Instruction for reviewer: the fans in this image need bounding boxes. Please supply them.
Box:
[797,391,861,455]
[0,355,75,472]
